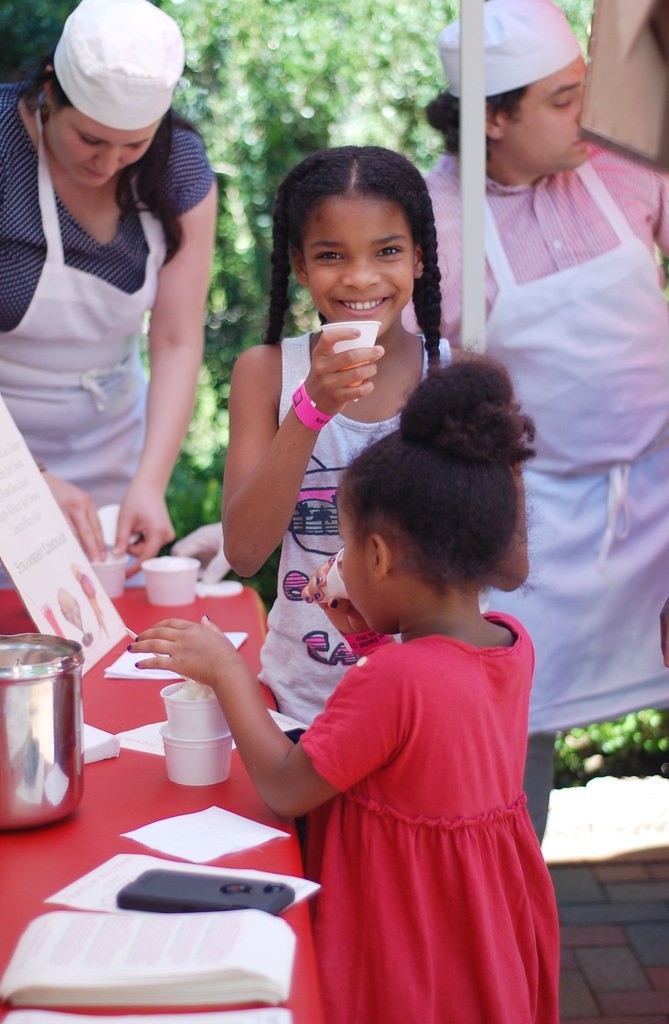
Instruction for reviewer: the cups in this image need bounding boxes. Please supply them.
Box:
[305,548,351,605]
[320,321,383,401]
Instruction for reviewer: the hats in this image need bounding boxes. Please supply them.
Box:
[436,0,582,98]
[54,0,186,131]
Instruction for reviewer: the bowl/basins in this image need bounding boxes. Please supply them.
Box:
[89,551,129,599]
[160,681,232,740]
[140,556,200,606]
[160,723,233,787]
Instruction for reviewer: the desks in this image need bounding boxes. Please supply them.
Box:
[0,578,331,1024]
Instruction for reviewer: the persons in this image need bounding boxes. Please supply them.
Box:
[224,147,480,840]
[423,2,669,749]
[0,1,218,600]
[127,355,559,1023]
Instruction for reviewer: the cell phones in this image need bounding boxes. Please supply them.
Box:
[118,868,295,916]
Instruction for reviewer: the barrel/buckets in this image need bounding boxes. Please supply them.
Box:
[1,633,86,830]
